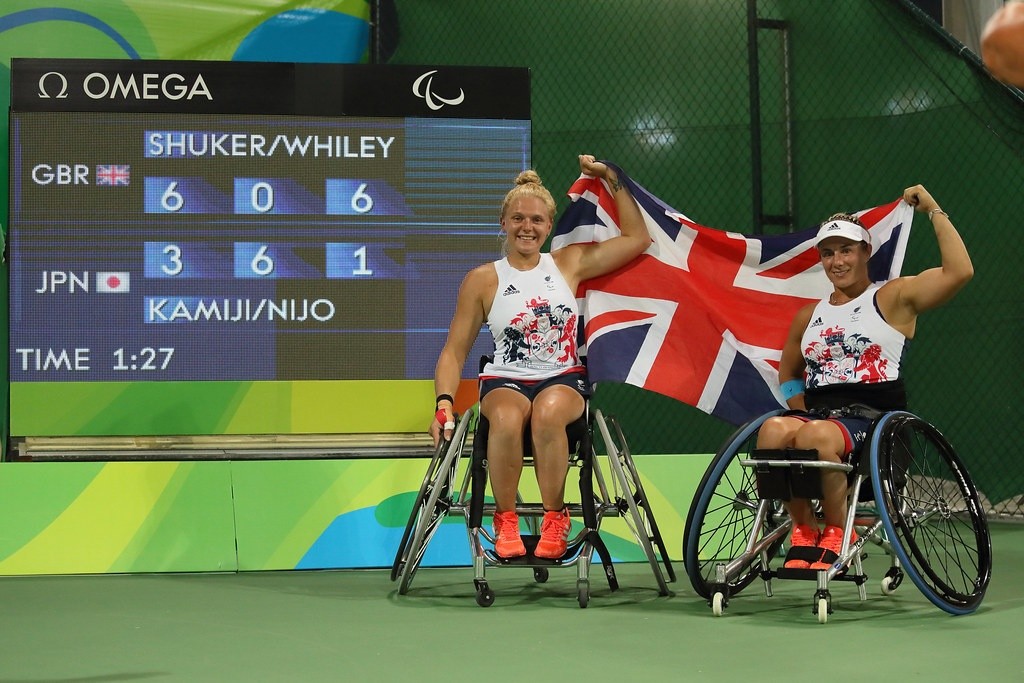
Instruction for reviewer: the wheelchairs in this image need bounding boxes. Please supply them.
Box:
[390,409,678,609]
[681,407,993,624]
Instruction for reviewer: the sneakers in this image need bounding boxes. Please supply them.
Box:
[783,523,823,569]
[491,508,527,559]
[533,506,573,559]
[809,525,858,571]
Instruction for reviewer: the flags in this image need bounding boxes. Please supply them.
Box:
[549,159,915,426]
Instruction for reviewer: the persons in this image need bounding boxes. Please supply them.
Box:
[428,154,652,561]
[758,183,973,569]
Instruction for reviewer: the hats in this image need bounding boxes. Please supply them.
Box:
[814,220,871,246]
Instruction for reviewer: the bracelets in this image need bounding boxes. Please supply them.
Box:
[435,394,453,405]
[927,208,948,220]
